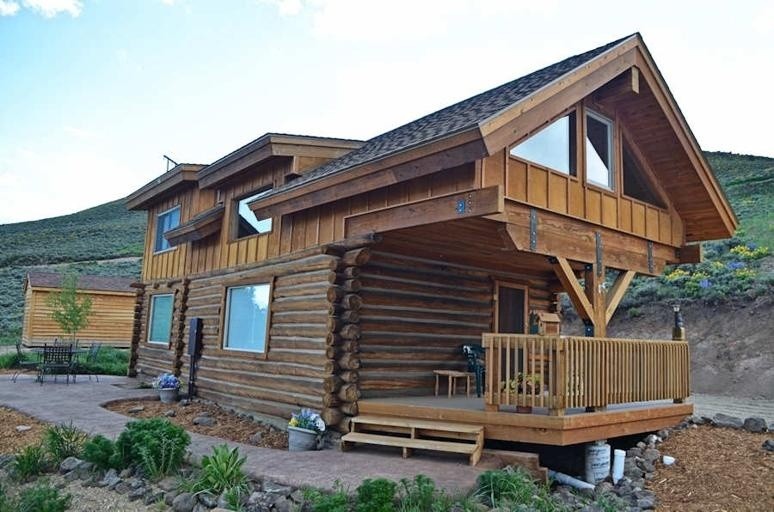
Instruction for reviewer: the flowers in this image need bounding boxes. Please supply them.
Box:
[151,372,184,389]
[289,407,326,433]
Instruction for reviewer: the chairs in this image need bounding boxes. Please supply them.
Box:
[12,334,101,387]
[460,344,486,398]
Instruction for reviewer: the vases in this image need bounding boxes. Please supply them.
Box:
[157,389,180,404]
[288,424,319,451]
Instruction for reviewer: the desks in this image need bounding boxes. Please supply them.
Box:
[433,369,470,399]
